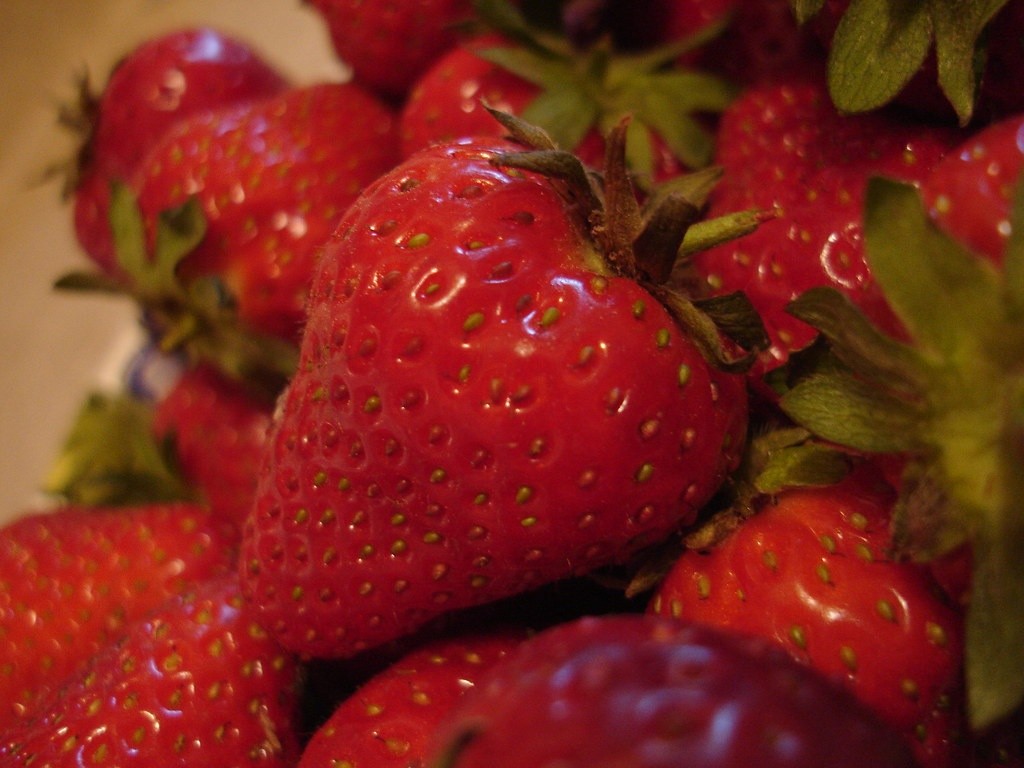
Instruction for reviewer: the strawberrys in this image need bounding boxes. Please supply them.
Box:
[0,0,1024,768]
